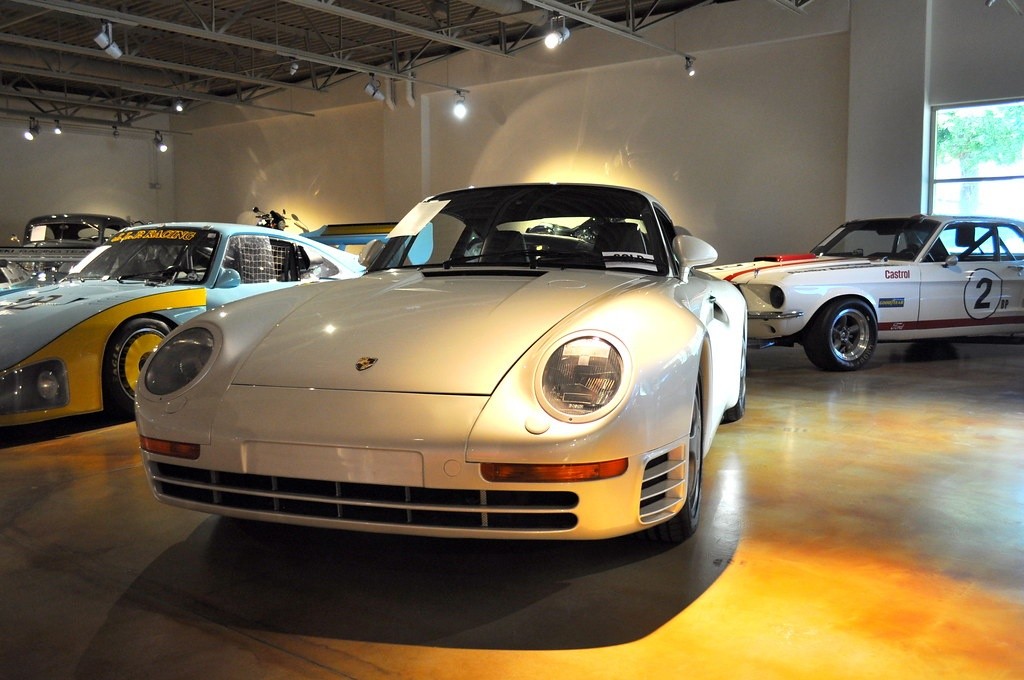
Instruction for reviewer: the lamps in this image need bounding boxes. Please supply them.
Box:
[363,72,386,102]
[152,131,167,153]
[94,22,123,59]
[453,91,465,119]
[24,118,42,140]
[289,62,299,76]
[176,99,183,111]
[544,11,570,48]
[53,120,62,134]
[684,56,696,76]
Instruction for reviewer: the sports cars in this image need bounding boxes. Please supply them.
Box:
[133,183,750,553]
[0,221,366,428]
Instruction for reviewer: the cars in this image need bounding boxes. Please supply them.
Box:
[297,220,398,253]
[694,215,1023,372]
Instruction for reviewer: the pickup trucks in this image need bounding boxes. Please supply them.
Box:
[0,212,145,284]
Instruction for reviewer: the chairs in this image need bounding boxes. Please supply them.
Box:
[474,230,531,264]
[593,222,648,257]
